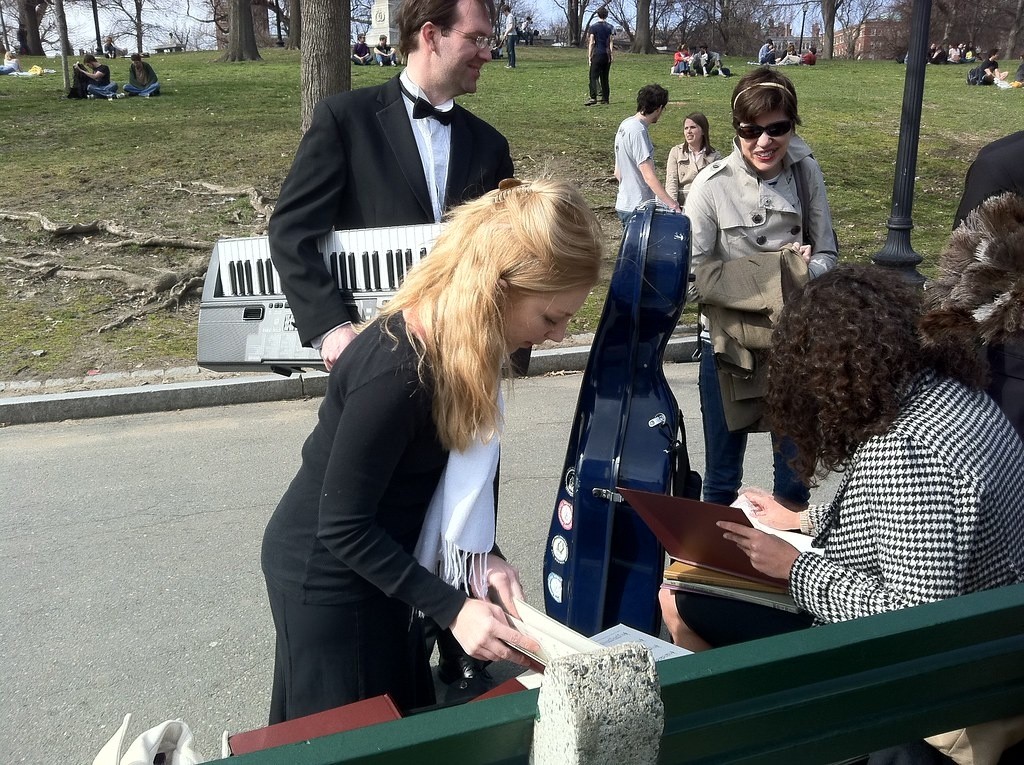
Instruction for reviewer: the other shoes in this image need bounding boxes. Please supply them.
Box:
[504,65,514,69]
[600,99,610,105]
[391,63,396,67]
[583,98,598,106]
[378,62,383,67]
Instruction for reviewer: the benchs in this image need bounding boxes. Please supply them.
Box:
[195,581,1024,765]
[150,43,186,54]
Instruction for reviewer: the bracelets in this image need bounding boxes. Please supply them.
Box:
[800,511,809,534]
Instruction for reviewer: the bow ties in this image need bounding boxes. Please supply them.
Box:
[395,79,456,127]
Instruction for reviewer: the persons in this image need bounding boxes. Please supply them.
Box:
[904,41,980,65]
[73,54,126,99]
[758,38,816,65]
[351,33,374,65]
[682,64,837,510]
[673,43,727,78]
[261,156,666,728]
[123,53,160,96]
[169,33,183,51]
[613,81,724,213]
[968,49,1009,86]
[267,0,531,697]
[103,36,116,59]
[490,5,533,68]
[659,257,1024,653]
[374,35,397,67]
[951,127,1024,437]
[1015,55,1024,88]
[584,7,617,105]
[0,51,22,75]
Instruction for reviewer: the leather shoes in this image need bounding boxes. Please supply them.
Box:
[437,646,498,691]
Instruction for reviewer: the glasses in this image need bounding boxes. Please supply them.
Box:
[361,37,365,39]
[427,17,497,50]
[732,120,794,140]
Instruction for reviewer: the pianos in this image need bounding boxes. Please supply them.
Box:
[193,219,533,381]
